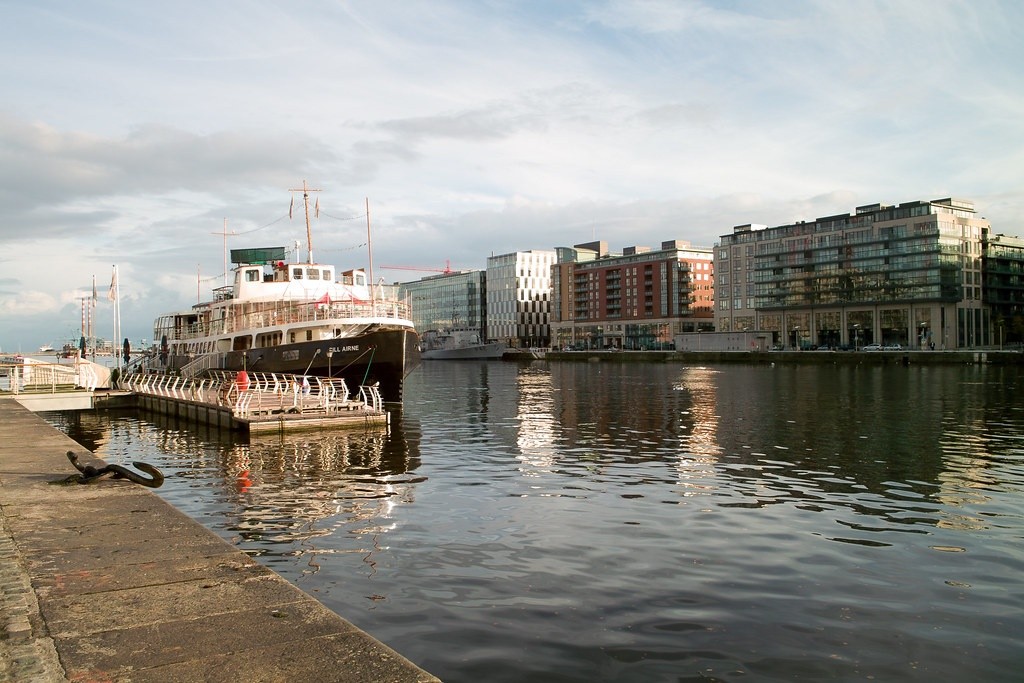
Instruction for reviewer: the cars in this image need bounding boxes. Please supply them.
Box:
[863,344,884,351]
[562,345,584,351]
[884,344,904,351]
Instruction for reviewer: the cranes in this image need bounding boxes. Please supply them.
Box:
[380,259,452,274]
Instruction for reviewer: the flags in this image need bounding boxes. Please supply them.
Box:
[289,196,294,219]
[93,279,97,307]
[107,271,116,301]
[315,196,319,218]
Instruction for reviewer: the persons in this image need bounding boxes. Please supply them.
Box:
[111,369,120,390]
[132,364,142,373]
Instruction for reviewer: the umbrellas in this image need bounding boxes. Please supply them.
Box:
[80,337,86,358]
[161,336,168,360]
[123,338,131,363]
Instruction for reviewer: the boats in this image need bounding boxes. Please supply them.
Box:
[141,179,422,414]
